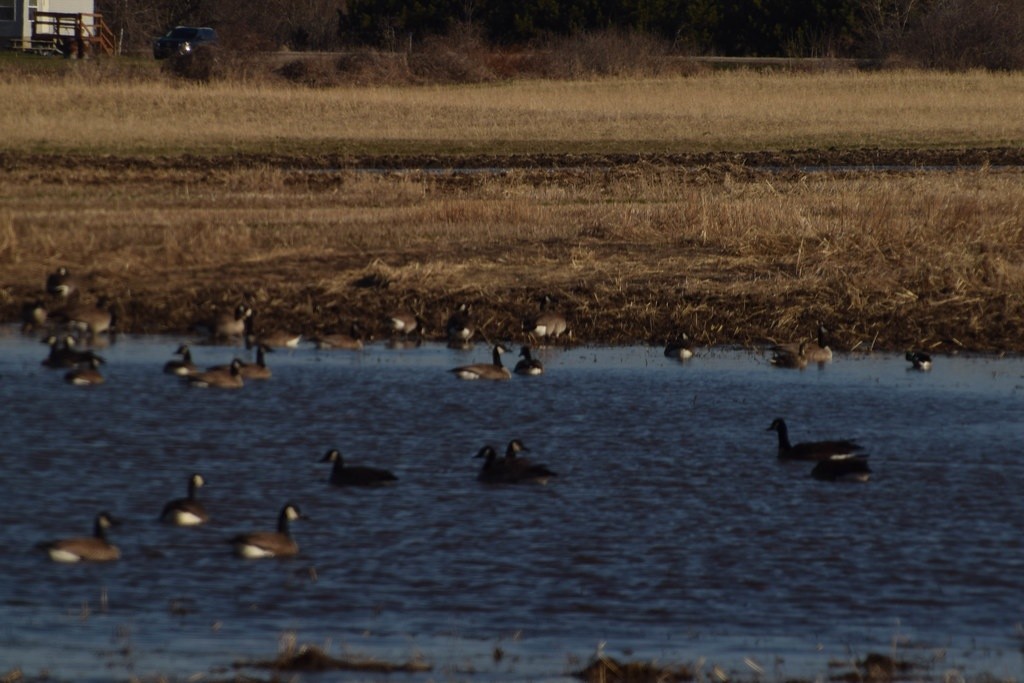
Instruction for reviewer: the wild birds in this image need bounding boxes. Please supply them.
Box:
[35,512,122,563]
[474,438,558,485]
[25,267,116,385]
[664,331,694,359]
[308,296,475,347]
[318,449,399,488]
[905,349,932,371]
[769,324,832,369]
[227,503,309,560]
[447,294,567,380]
[765,418,872,483]
[161,473,211,527]
[164,305,300,388]
